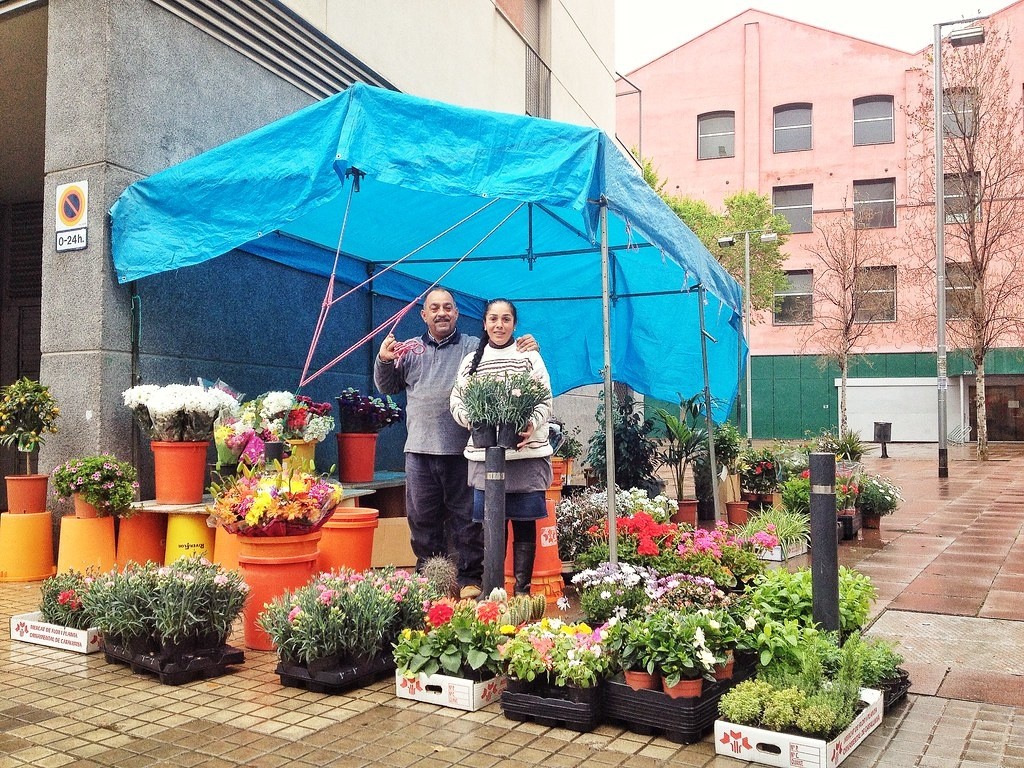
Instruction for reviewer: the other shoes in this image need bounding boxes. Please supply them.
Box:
[459,585,482,599]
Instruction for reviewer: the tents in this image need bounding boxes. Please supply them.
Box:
[106,80,750,578]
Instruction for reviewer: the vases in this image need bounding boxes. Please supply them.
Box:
[43,433,909,755]
[497,421,527,450]
[470,419,496,448]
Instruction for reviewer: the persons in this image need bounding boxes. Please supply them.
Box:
[374,287,539,597]
[449,299,553,600]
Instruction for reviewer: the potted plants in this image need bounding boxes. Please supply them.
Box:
[0,376,59,512]
[633,384,728,531]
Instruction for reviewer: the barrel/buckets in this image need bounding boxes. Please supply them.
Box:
[502,499,566,604]
[329,507,379,522]
[115,513,166,571]
[164,514,215,568]
[0,512,57,582]
[318,521,378,576]
[212,519,239,571]
[56,515,117,578]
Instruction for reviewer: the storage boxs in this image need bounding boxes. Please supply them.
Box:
[398,668,512,712]
[714,680,884,768]
[8,611,113,654]
[753,538,808,564]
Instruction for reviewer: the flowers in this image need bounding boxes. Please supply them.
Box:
[454,362,549,415]
[648,572,731,614]
[77,550,260,618]
[855,471,910,514]
[555,557,659,612]
[624,604,760,676]
[388,591,517,664]
[551,476,680,548]
[253,554,433,654]
[48,450,145,520]
[202,455,348,535]
[546,418,581,459]
[792,464,861,504]
[238,390,337,447]
[213,408,253,447]
[339,387,397,433]
[119,378,245,425]
[670,518,784,570]
[492,610,625,686]
[588,511,683,559]
[739,444,778,484]
[38,558,104,620]
